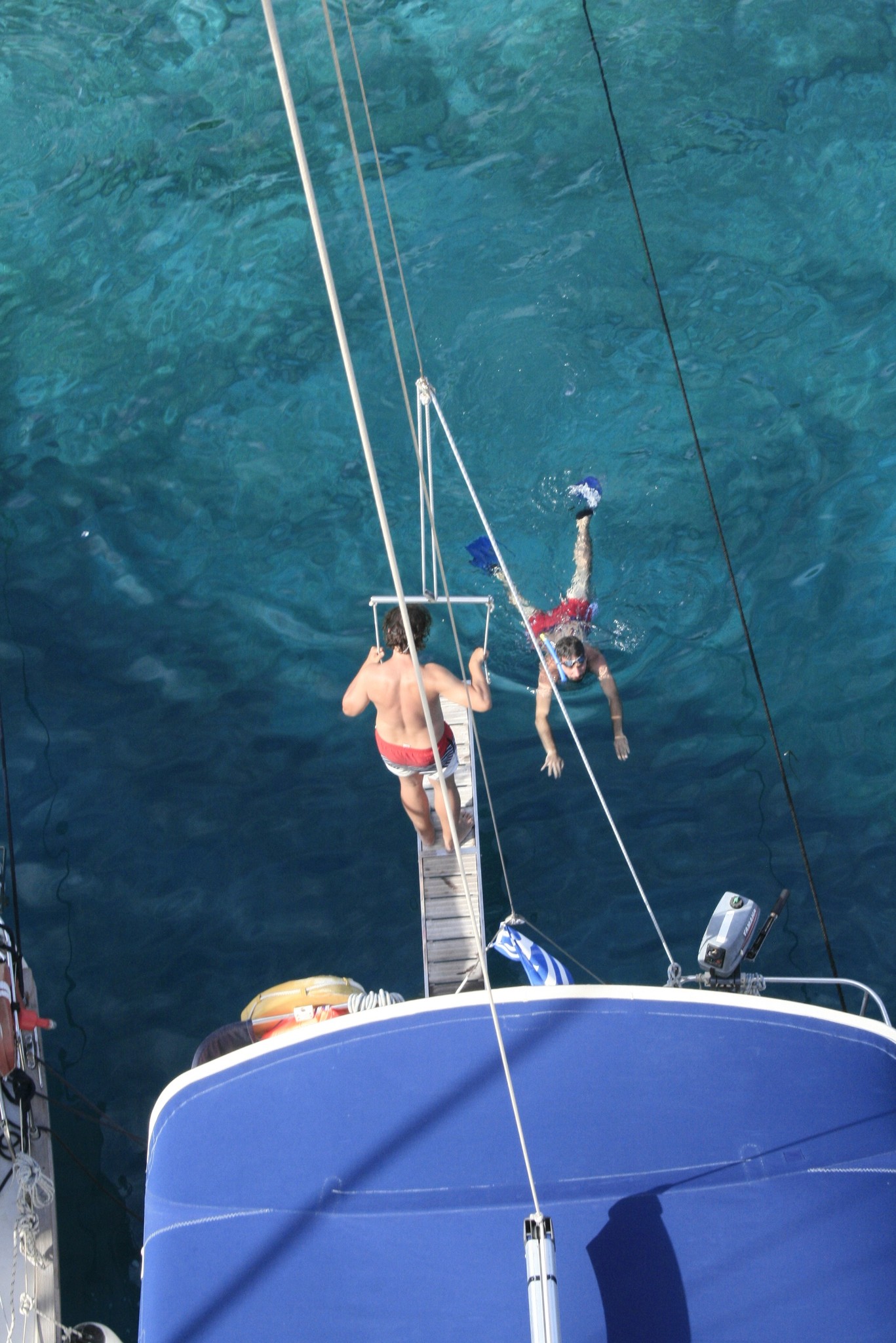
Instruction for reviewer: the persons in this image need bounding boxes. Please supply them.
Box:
[485,506,630,780]
[341,603,493,853]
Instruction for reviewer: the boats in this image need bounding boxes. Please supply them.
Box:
[0,707,61,1343]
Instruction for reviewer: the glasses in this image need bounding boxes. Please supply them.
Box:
[562,655,586,667]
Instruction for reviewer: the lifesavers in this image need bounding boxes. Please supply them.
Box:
[233,974,370,1033]
[0,962,20,1079]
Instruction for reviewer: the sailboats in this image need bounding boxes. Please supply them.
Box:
[141,0,896,1343]
